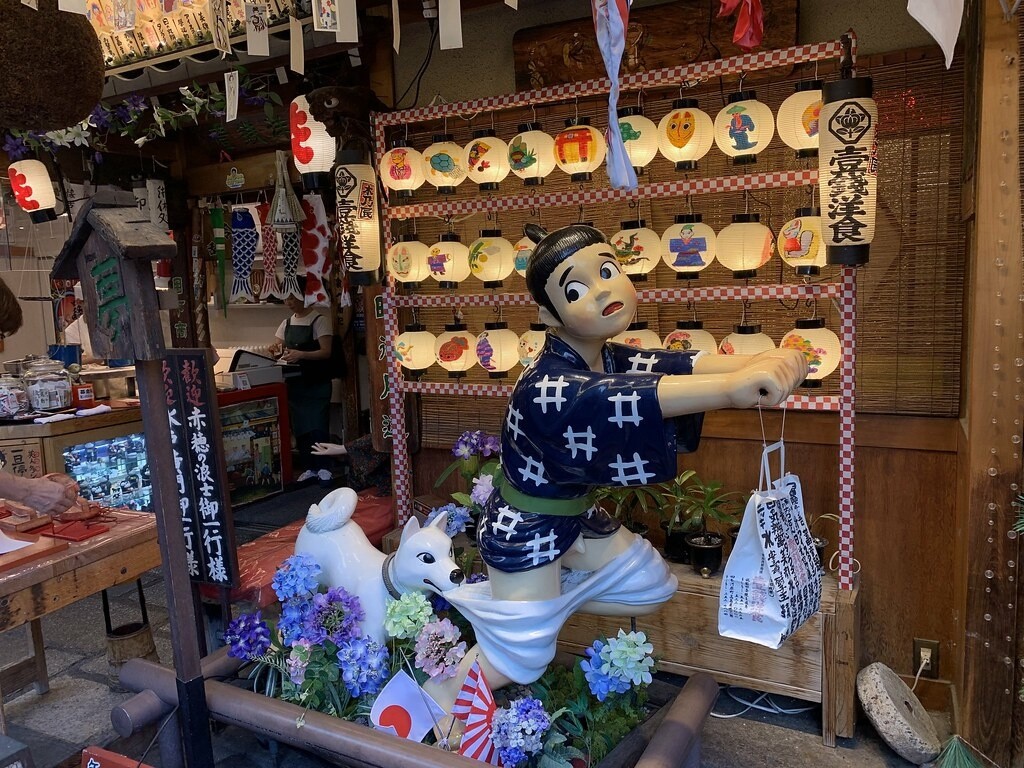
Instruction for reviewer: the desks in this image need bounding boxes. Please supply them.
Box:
[0,499,165,737]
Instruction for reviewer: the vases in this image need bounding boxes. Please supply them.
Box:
[463,510,484,549]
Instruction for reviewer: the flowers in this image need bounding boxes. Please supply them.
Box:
[433,427,501,517]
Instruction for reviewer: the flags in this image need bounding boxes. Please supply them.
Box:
[369,669,447,745]
[451,660,504,767]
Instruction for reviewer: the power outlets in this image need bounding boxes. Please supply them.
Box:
[913,636,940,680]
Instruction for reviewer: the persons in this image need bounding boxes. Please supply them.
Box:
[0,278,77,517]
[422,223,812,762]
[310,431,393,498]
[62,280,136,403]
[267,296,339,483]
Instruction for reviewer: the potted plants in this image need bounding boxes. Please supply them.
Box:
[650,468,723,564]
[593,480,673,541]
[804,512,840,576]
[726,488,759,550]
[667,471,744,577]
